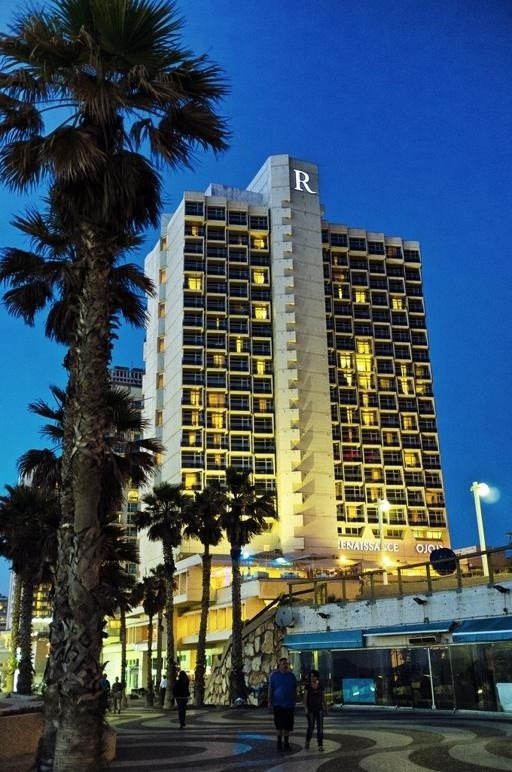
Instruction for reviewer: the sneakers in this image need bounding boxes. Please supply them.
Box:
[276,742,292,754]
[305,741,310,748]
[318,746,323,751]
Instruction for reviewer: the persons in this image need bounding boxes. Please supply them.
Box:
[103,673,110,712]
[159,675,168,706]
[111,677,123,714]
[267,658,297,752]
[304,670,329,751]
[172,671,190,728]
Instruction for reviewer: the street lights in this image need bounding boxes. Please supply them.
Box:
[377,499,391,584]
[470,481,490,576]
